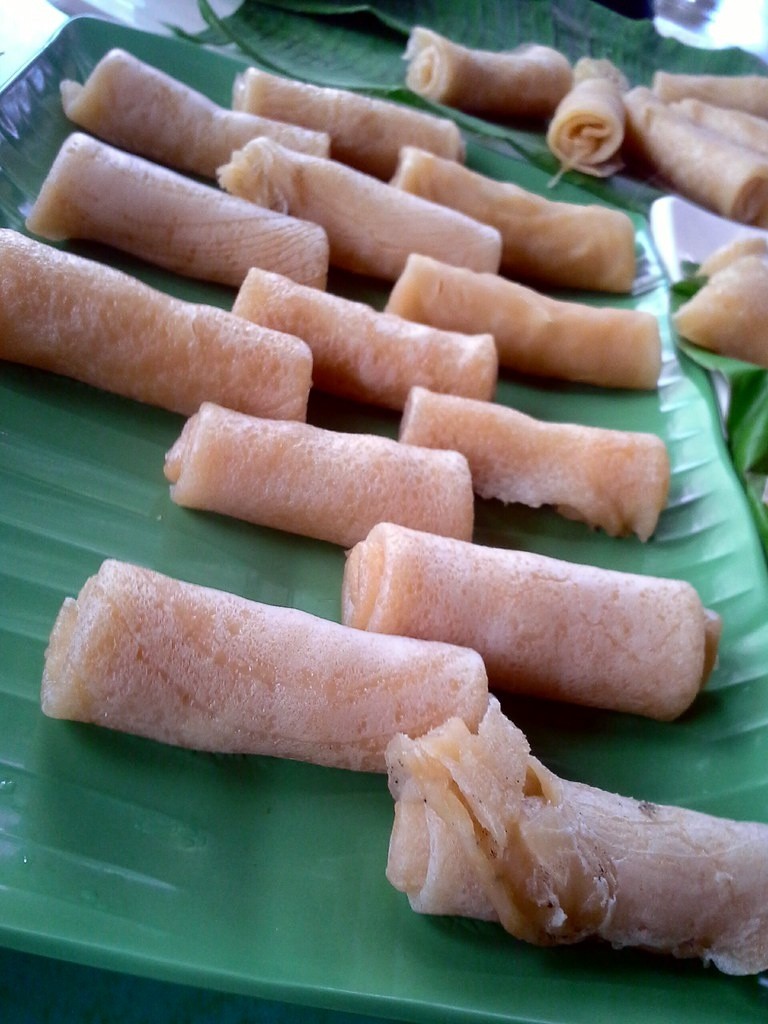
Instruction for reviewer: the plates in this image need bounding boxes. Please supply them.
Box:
[651,195,768,425]
[0,13,768,1024]
